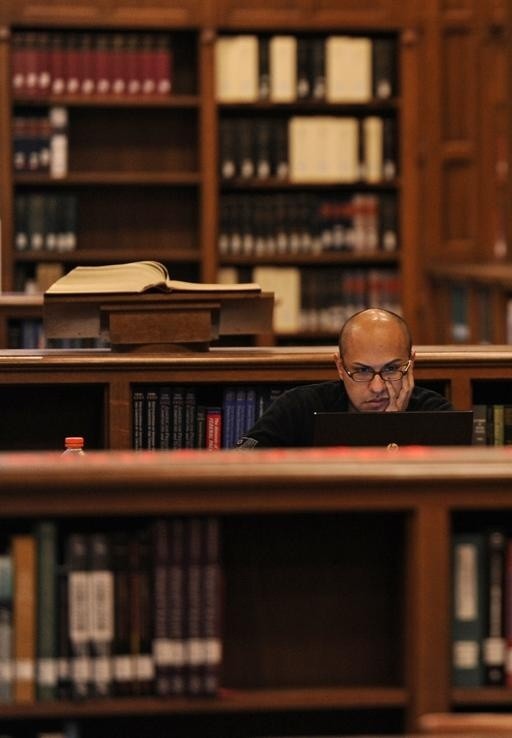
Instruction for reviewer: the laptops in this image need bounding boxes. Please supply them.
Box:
[313,410,474,445]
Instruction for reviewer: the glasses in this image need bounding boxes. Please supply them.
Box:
[339,351,413,382]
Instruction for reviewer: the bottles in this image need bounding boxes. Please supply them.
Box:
[55,434,90,456]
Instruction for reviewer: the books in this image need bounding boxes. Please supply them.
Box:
[472,405,511,445]
[11,30,173,96]
[449,524,512,689]
[1,515,223,706]
[217,44,404,332]
[131,389,284,451]
[12,118,111,349]
[46,261,262,294]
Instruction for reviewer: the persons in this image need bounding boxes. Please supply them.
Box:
[234,309,457,450]
